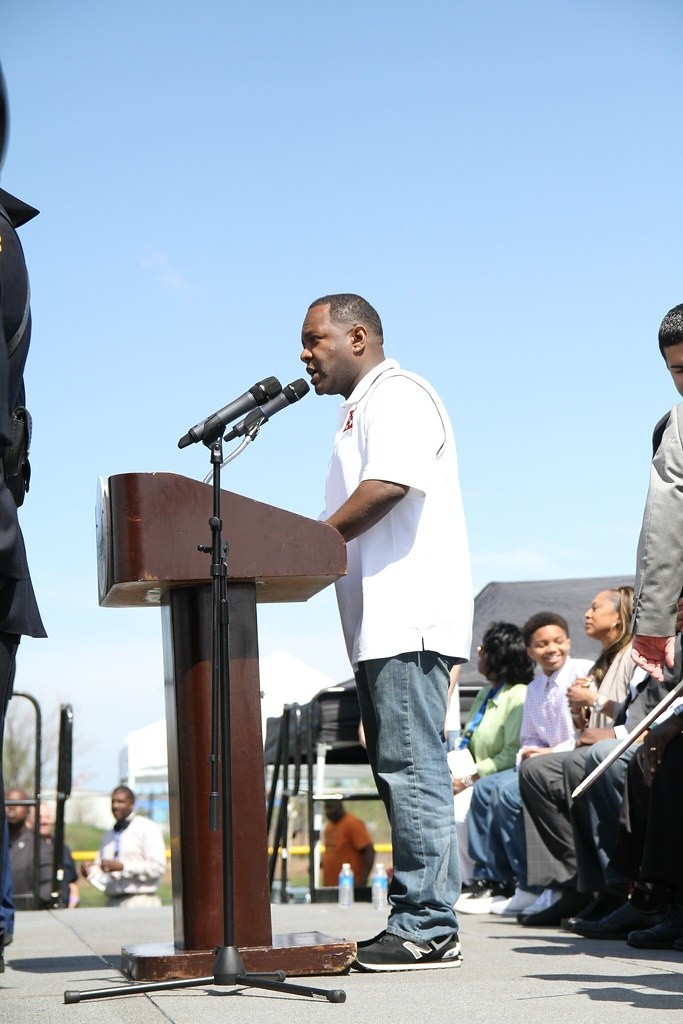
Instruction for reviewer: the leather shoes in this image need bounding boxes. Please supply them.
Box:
[561,900,625,929]
[573,901,668,938]
[522,895,596,925]
[627,921,683,949]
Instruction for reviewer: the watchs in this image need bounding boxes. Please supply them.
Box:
[593,695,607,712]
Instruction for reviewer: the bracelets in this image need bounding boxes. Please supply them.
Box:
[460,776,473,788]
[569,708,584,718]
[69,896,80,903]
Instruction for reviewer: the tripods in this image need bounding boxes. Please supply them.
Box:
[64,436,348,1005]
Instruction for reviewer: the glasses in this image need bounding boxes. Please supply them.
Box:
[477,645,486,656]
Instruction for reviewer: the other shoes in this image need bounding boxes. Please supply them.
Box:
[454,882,497,898]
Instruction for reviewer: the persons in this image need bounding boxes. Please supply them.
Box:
[445,586,683,950]
[300,294,476,973]
[79,786,167,908]
[0,69,47,973]
[5,787,81,911]
[358,664,461,774]
[628,302,683,681]
[320,799,376,888]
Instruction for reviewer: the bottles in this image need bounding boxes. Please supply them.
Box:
[371,864,387,910]
[338,863,354,906]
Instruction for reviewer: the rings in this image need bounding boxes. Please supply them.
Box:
[649,747,656,751]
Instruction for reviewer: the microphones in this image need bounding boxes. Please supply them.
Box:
[224,378,310,442]
[177,377,283,450]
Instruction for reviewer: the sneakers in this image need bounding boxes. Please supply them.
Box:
[350,927,464,972]
[453,893,506,914]
[522,888,564,914]
[490,887,539,915]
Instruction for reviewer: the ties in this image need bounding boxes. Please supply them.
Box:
[114,825,120,860]
